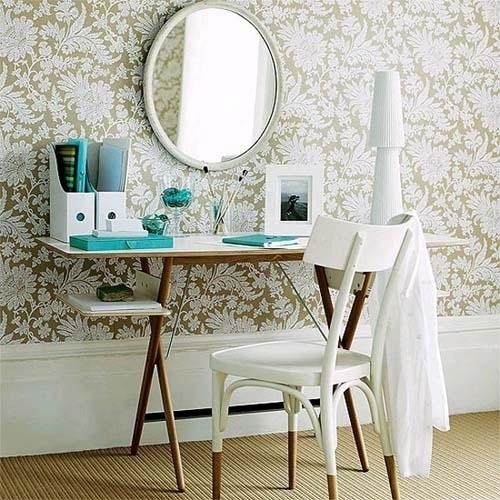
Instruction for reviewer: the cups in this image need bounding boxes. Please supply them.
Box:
[211,198,234,234]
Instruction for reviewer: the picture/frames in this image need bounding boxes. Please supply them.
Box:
[263,161,325,237]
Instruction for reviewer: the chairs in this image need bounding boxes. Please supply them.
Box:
[208,213,423,499]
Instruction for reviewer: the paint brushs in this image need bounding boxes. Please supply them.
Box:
[203,166,248,235]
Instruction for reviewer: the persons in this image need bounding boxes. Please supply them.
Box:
[286,195,300,216]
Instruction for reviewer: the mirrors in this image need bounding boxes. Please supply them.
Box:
[142,0,285,171]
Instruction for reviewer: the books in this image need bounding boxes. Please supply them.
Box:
[222,234,301,247]
[54,136,89,221]
[92,230,148,239]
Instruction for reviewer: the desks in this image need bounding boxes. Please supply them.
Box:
[31,229,469,493]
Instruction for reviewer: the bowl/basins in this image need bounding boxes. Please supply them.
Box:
[138,217,171,236]
